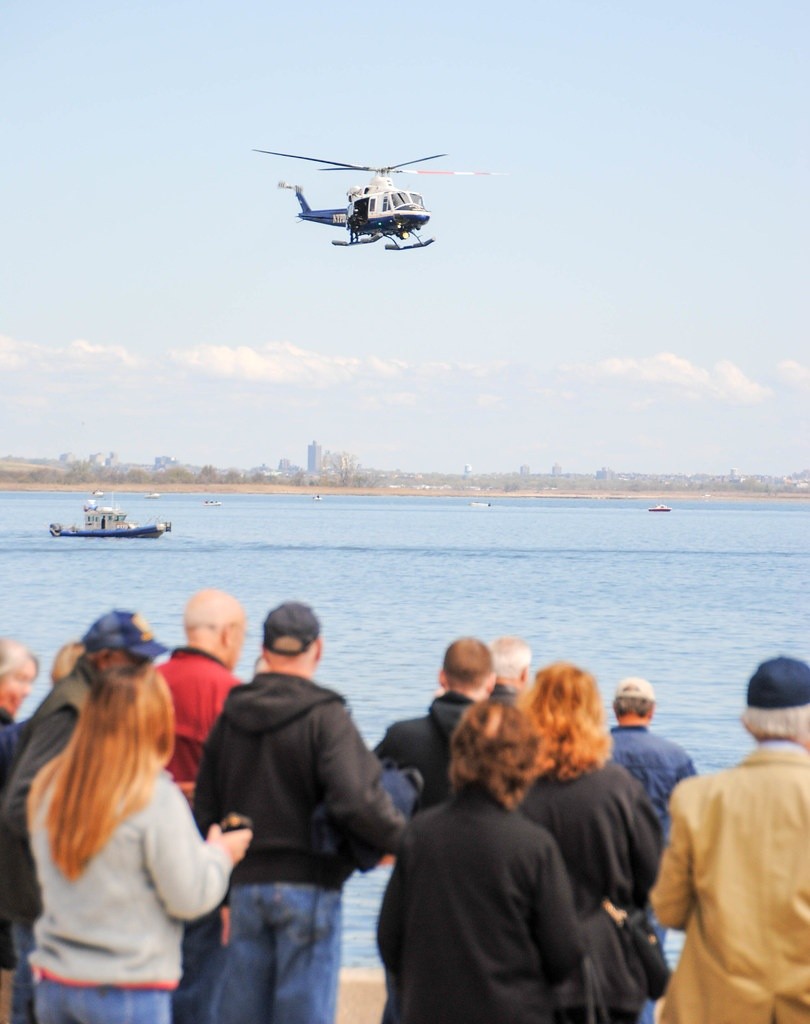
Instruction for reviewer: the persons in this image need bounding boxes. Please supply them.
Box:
[612,676,698,1024]
[194,603,404,1024]
[377,701,577,1024]
[25,665,253,1024]
[373,639,500,815]
[649,657,810,1024]
[488,635,532,705]
[153,587,250,1024]
[518,661,663,914]
[0,610,168,1024]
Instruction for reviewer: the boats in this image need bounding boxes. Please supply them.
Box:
[202,500,222,506]
[50,500,173,538]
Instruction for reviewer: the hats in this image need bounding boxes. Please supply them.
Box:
[83,611,168,657]
[617,678,655,701]
[264,603,321,655]
[747,656,810,708]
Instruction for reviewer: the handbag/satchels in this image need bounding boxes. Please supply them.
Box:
[620,913,669,1001]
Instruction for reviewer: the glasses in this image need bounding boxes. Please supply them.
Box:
[113,653,146,665]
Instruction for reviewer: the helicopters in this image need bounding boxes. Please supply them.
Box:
[250,148,507,251]
